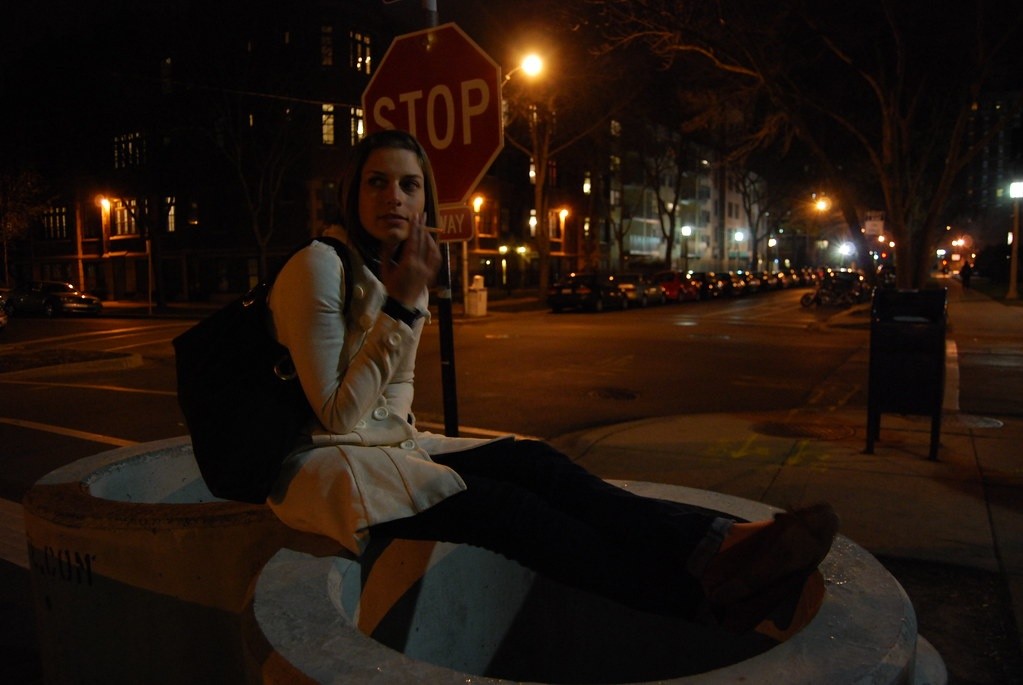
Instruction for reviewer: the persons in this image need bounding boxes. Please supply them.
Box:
[959,260,972,291]
[263,128,838,634]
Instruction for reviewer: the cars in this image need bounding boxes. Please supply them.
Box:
[5,278,103,318]
[551,272,629,313]
[658,269,796,300]
[616,273,670,306]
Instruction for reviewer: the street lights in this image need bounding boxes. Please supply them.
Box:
[518,51,547,187]
[1007,182,1022,303]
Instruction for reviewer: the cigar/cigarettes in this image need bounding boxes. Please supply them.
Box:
[418,226,442,233]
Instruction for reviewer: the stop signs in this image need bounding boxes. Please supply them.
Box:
[360,24,504,215]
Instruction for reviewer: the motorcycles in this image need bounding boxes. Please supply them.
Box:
[799,265,895,311]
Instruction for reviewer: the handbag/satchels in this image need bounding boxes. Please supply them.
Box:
[171,239,353,504]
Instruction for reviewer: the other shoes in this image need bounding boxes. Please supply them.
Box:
[703,506,839,641]
